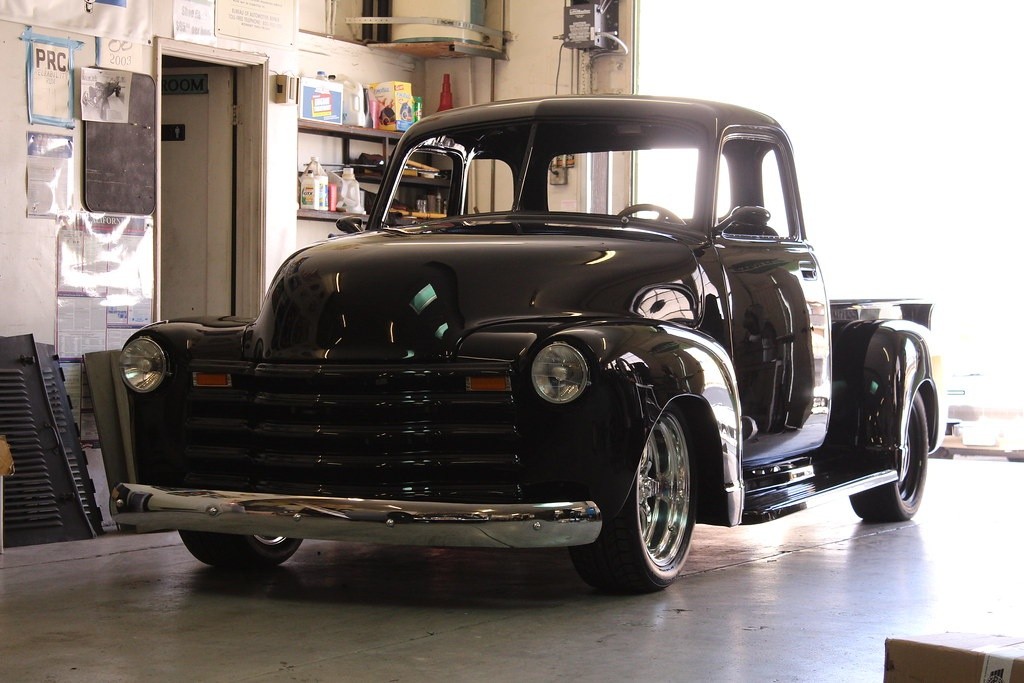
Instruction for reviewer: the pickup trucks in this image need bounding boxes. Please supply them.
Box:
[108,90,942,595]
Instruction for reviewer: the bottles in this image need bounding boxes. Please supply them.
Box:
[300,171,318,210]
[341,168,360,205]
[302,155,329,211]
[436,74,453,111]
[316,71,366,127]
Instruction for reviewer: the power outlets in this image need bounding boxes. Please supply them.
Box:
[550,168,567,185]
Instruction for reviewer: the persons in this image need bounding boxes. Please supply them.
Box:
[742,303,778,410]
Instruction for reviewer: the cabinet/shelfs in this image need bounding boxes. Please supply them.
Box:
[297,119,451,226]
